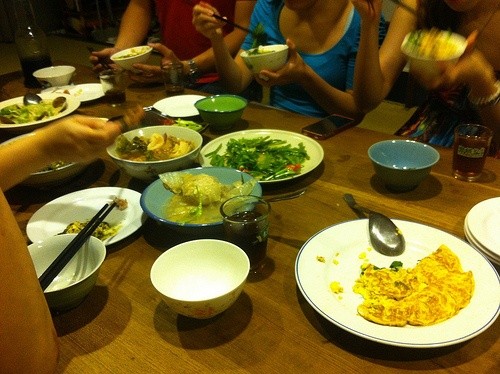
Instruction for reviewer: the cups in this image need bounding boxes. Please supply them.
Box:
[160,63,186,94]
[99,69,126,104]
[452,125,493,183]
[220,194,271,275]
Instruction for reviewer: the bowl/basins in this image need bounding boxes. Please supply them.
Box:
[195,95,248,133]
[149,240,250,320]
[141,166,265,235]
[109,46,153,70]
[34,65,77,89]
[0,95,81,132]
[240,44,290,75]
[105,126,205,181]
[20,163,87,187]
[401,30,468,80]
[369,139,440,193]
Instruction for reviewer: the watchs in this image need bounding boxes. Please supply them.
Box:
[187,59,199,78]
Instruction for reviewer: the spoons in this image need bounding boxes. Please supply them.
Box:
[343,193,406,257]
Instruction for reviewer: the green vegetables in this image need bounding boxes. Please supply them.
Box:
[205,136,309,180]
[0,103,60,124]
[247,25,271,55]
[404,28,424,54]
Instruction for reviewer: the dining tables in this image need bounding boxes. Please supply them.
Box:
[0,57,500,374]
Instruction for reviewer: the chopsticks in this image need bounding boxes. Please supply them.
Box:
[88,46,113,72]
[38,201,116,291]
[214,12,282,44]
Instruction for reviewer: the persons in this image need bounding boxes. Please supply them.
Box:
[192,0,386,124]
[89,0,252,95]
[351,0,500,160]
[0,116,122,374]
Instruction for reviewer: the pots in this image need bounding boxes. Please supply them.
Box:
[27,234,106,315]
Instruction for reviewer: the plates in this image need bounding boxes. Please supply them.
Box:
[150,94,203,117]
[27,185,145,246]
[462,196,500,266]
[294,219,500,348]
[50,83,109,102]
[197,128,324,184]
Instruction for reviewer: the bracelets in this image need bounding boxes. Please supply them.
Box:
[469,81,500,106]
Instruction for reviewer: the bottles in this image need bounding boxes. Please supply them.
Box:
[10,0,51,87]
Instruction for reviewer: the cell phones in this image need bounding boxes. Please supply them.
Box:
[302,113,355,141]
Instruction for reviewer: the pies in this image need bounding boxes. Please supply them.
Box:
[353,244,475,327]
